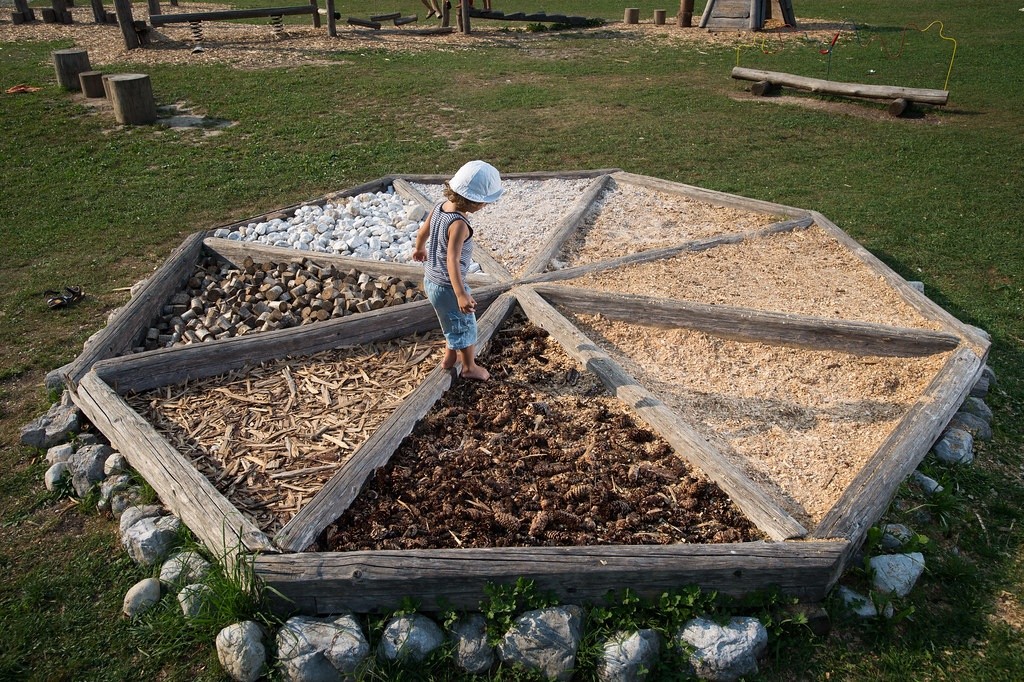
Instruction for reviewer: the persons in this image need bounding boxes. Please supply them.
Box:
[412,160,506,382]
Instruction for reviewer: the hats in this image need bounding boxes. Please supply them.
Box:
[448,160,507,203]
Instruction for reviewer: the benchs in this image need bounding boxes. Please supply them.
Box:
[732,66,950,118]
